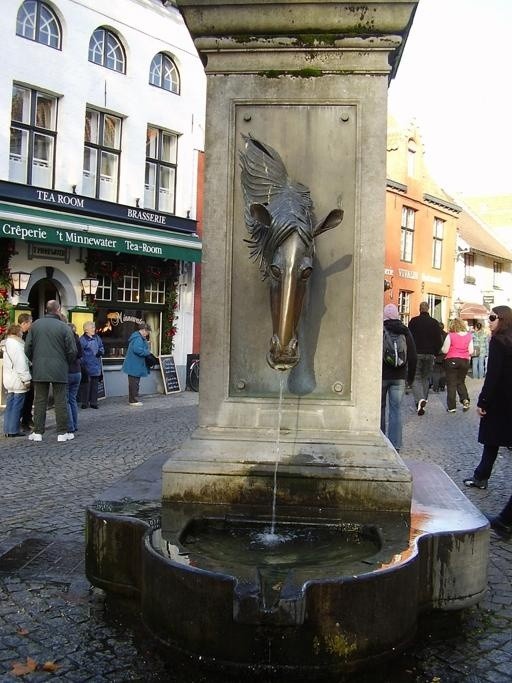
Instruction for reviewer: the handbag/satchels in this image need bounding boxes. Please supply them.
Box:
[81,367,90,384]
[145,353,159,366]
[383,327,407,369]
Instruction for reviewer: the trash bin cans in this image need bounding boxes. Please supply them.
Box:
[185,354,199,391]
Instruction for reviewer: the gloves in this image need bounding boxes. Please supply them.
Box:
[96,350,102,358]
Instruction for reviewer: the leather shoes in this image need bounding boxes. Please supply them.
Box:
[82,404,86,409]
[91,404,99,409]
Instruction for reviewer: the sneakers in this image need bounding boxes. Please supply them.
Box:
[462,477,487,490]
[418,399,427,416]
[463,400,471,408]
[28,432,42,442]
[130,401,143,406]
[58,433,74,441]
[483,511,510,536]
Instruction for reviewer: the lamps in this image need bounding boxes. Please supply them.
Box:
[81,278,100,301]
[10,271,32,297]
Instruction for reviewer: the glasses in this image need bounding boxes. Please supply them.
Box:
[489,315,498,321]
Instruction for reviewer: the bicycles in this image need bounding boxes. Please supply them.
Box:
[189,358,200,392]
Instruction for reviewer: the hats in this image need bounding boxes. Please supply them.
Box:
[383,304,398,321]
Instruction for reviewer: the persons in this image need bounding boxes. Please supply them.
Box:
[24,296,78,441]
[77,318,105,410]
[429,321,448,393]
[380,303,418,455]
[16,312,35,429]
[0,322,33,438]
[470,321,486,379]
[120,322,160,406]
[406,300,444,417]
[439,315,475,412]
[58,310,85,434]
[462,302,512,491]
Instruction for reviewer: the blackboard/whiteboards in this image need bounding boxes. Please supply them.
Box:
[159,355,182,395]
[97,355,106,401]
